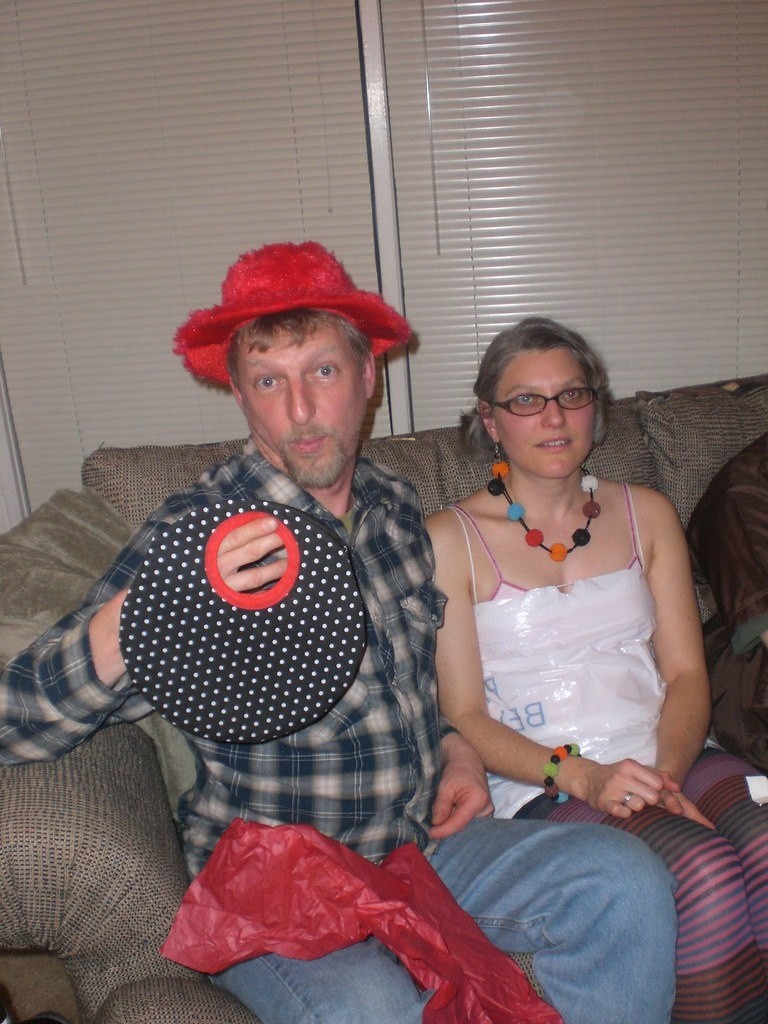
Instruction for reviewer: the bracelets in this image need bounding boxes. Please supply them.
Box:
[545,743,581,804]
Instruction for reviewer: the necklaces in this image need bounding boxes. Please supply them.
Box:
[487,462,600,560]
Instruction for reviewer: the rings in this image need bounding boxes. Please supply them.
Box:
[622,792,631,806]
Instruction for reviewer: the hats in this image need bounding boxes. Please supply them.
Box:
[118,499,367,745]
[171,239,411,388]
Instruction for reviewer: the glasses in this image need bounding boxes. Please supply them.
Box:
[490,387,597,416]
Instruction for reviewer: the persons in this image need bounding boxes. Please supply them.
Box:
[0,242,680,1023]
[687,432,767,776]
[426,313,768,1024]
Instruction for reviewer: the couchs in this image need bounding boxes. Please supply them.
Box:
[0,373,768,1024]
